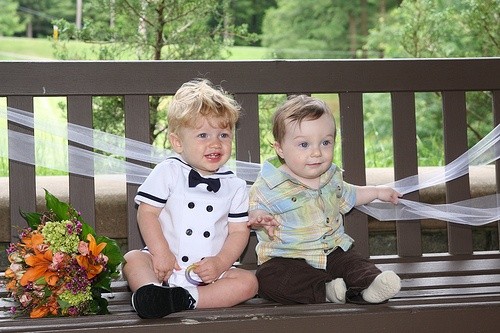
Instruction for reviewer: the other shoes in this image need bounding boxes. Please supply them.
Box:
[132,284,196,320]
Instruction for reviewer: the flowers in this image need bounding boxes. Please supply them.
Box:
[0,189,128,320]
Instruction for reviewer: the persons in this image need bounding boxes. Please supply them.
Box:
[123,82,259,320]
[248,95,401,304]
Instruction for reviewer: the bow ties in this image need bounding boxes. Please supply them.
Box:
[189,168,221,193]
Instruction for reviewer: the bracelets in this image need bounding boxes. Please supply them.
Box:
[185,265,206,286]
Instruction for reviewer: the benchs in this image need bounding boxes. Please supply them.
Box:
[0,58,500,333]
